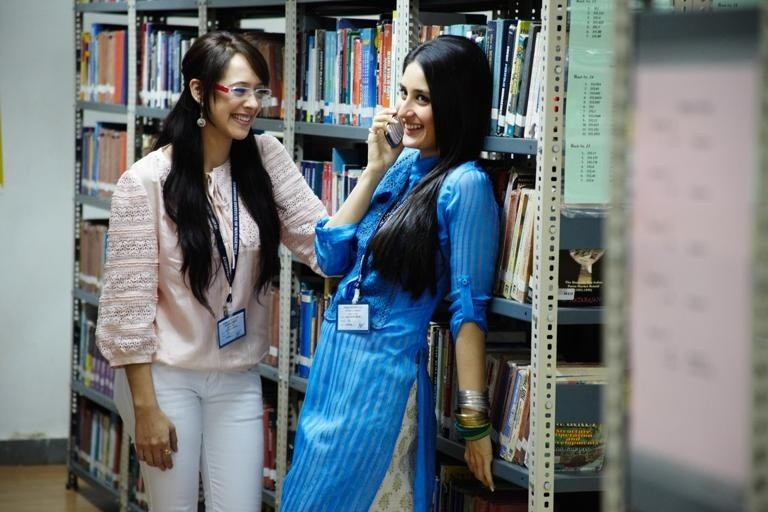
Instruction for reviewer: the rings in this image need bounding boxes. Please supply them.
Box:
[163,448,171,456]
[368,128,377,134]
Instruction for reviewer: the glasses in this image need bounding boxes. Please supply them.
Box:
[211,82,273,97]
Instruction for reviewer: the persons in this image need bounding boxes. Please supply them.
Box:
[278,35,500,505]
[96,31,344,508]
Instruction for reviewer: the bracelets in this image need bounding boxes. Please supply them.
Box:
[456,390,491,442]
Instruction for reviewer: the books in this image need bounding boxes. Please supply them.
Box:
[80,27,195,110]
[263,270,340,378]
[230,28,283,118]
[129,441,206,507]
[427,320,604,472]
[489,164,602,307]
[73,298,116,398]
[80,220,109,295]
[411,18,570,162]
[77,395,122,488]
[263,396,305,489]
[434,465,600,508]
[82,122,159,186]
[299,143,366,218]
[299,16,393,128]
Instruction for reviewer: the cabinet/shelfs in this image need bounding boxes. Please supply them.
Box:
[66,0,634,512]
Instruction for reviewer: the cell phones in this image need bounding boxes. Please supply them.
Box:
[384,112,404,148]
